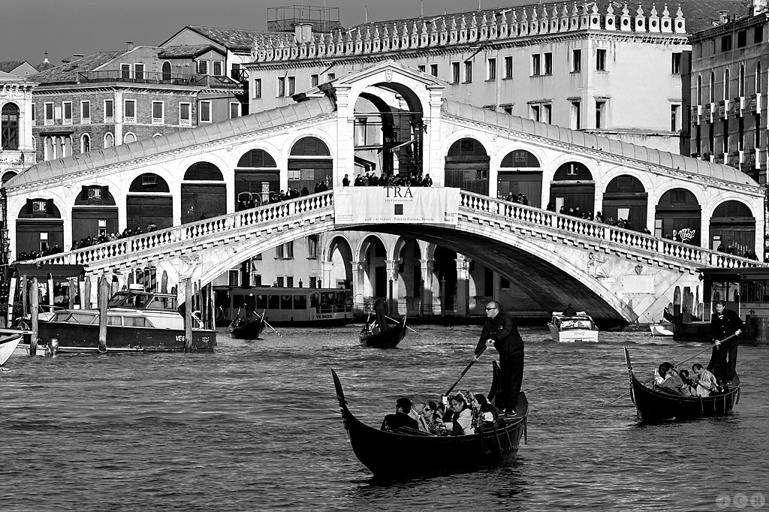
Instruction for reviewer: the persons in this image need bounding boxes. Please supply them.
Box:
[237,172,432,211]
[506,192,651,235]
[658,362,719,397]
[243,293,256,318]
[471,300,525,418]
[709,300,744,382]
[16,222,157,261]
[363,298,388,335]
[717,244,759,261]
[562,303,576,316]
[380,392,498,435]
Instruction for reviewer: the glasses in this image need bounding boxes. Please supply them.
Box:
[485,306,497,311]
[424,408,430,412]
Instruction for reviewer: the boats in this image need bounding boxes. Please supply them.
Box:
[623,267,769,422]
[546,311,599,343]
[331,360,529,475]
